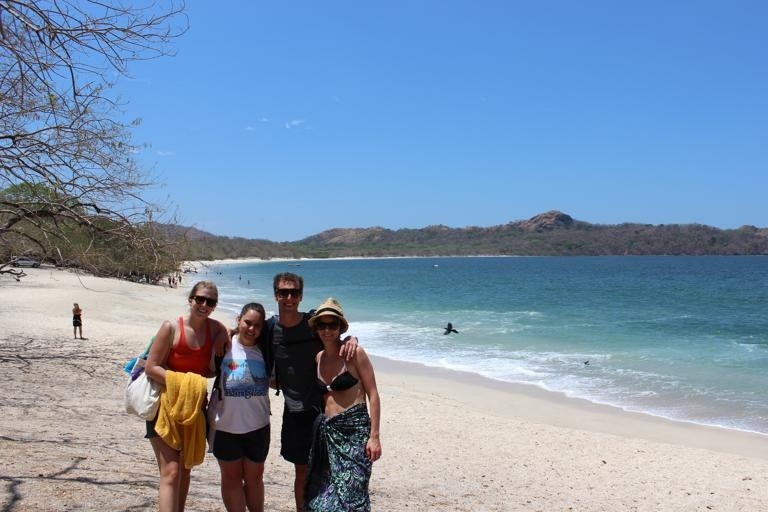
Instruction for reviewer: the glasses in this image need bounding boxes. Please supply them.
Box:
[315,321,339,330]
[191,296,217,307]
[276,289,302,297]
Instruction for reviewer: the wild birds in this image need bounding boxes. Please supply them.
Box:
[442,322,460,334]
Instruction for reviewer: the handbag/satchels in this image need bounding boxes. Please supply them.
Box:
[125,357,160,422]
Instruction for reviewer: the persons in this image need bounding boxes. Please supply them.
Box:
[215,273,358,512]
[168,267,250,288]
[73,304,84,339]
[210,302,280,512]
[303,298,381,512]
[144,281,225,512]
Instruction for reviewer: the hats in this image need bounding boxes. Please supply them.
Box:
[307,297,348,334]
